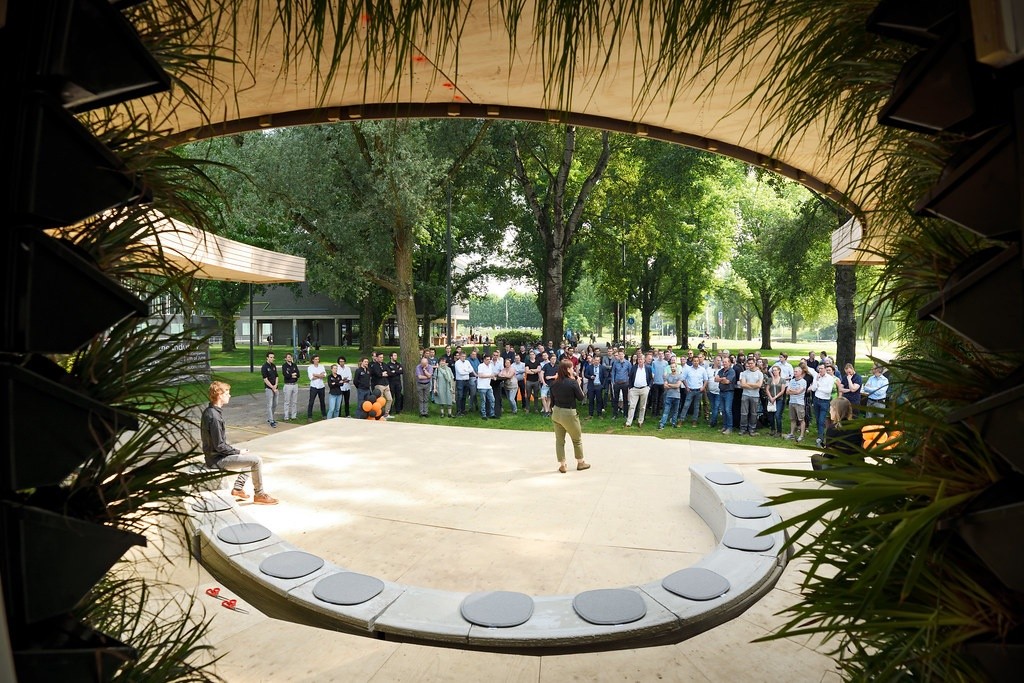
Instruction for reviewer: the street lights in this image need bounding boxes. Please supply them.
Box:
[868,313,875,357]
[735,318,740,340]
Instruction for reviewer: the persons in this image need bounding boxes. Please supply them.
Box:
[308,355,327,423]
[337,356,353,417]
[353,357,371,419]
[564,329,598,347]
[550,360,590,473]
[327,364,344,419]
[342,334,348,348]
[415,340,905,444]
[472,333,488,345]
[811,397,864,485]
[261,352,278,428]
[300,335,312,361]
[268,333,273,348]
[368,351,404,418]
[282,352,300,421]
[200,381,279,505]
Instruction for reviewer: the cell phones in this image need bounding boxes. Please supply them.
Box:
[593,375,596,380]
[816,438,825,448]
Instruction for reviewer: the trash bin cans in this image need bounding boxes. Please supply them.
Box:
[711,342,718,349]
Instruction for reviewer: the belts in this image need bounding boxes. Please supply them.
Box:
[633,386,645,389]
[868,397,886,401]
[690,389,702,391]
[418,381,430,384]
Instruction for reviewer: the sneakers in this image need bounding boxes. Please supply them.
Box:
[231,489,250,498]
[253,494,279,504]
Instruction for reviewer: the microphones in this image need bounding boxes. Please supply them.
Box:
[573,372,578,380]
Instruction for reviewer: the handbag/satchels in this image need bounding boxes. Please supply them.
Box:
[767,398,776,412]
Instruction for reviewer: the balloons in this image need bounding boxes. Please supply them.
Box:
[360,388,386,421]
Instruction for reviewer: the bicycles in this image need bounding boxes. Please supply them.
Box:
[300,350,310,365]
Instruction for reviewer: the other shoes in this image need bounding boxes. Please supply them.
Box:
[308,418,313,422]
[482,417,487,420]
[285,419,289,422]
[420,414,429,418]
[322,416,327,420]
[577,461,590,470]
[559,464,568,473]
[268,419,277,423]
[290,417,297,420]
[441,413,445,417]
[270,422,276,428]
[447,414,456,418]
[490,415,499,419]
[385,414,394,418]
[511,400,827,444]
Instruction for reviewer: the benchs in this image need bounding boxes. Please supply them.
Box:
[182,459,790,656]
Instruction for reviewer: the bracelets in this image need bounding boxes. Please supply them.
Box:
[423,369,426,371]
